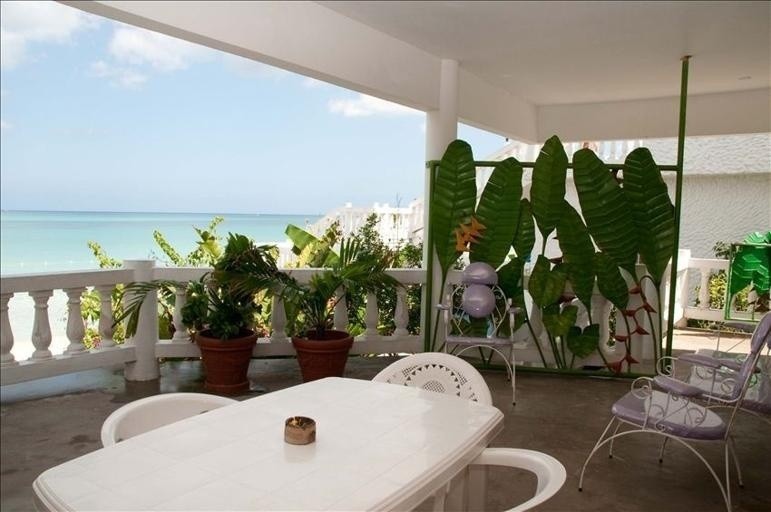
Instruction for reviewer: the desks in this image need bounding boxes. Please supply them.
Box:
[30,375,505,512]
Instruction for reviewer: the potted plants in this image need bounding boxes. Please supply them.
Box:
[265,228,410,391]
[109,228,309,391]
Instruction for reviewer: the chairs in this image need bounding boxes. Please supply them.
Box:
[438,260,524,405]
[99,350,567,512]
[577,311,771,512]
